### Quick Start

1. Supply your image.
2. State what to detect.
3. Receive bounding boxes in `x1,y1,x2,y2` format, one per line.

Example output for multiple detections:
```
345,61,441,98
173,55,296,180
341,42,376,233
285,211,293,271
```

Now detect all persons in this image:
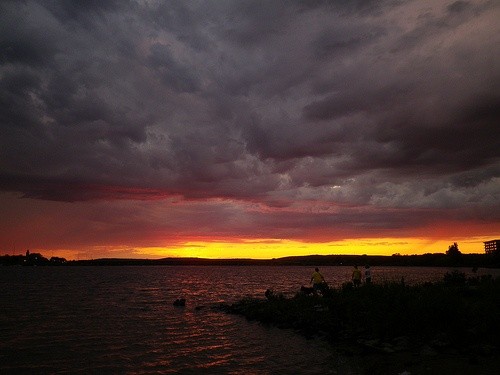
364,264,373,285
351,263,362,287
310,267,326,295
